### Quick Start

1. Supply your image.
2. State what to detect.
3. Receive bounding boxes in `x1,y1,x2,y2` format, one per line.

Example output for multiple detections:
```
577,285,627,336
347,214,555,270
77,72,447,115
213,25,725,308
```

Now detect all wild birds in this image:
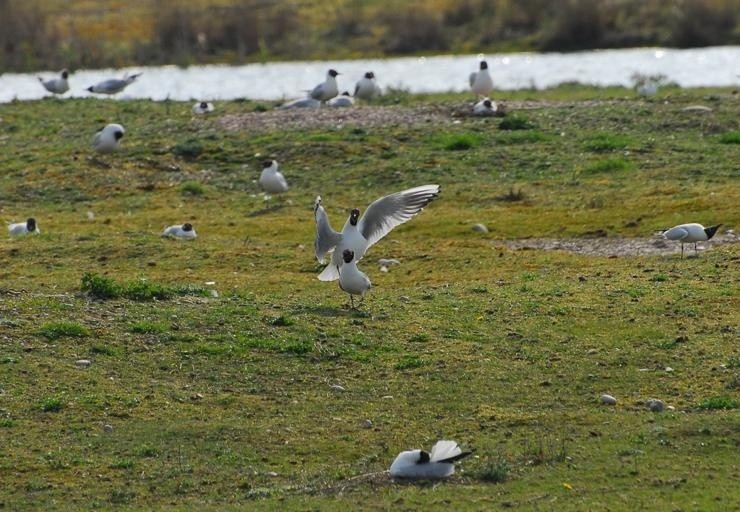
83,70,142,100
257,158,290,211
35,66,73,100
310,181,444,283
92,121,128,167
467,60,494,97
159,223,197,239
662,221,723,258
471,94,499,115
335,249,372,310
277,66,382,109
190,101,217,113
7,218,45,237
380,437,477,483
